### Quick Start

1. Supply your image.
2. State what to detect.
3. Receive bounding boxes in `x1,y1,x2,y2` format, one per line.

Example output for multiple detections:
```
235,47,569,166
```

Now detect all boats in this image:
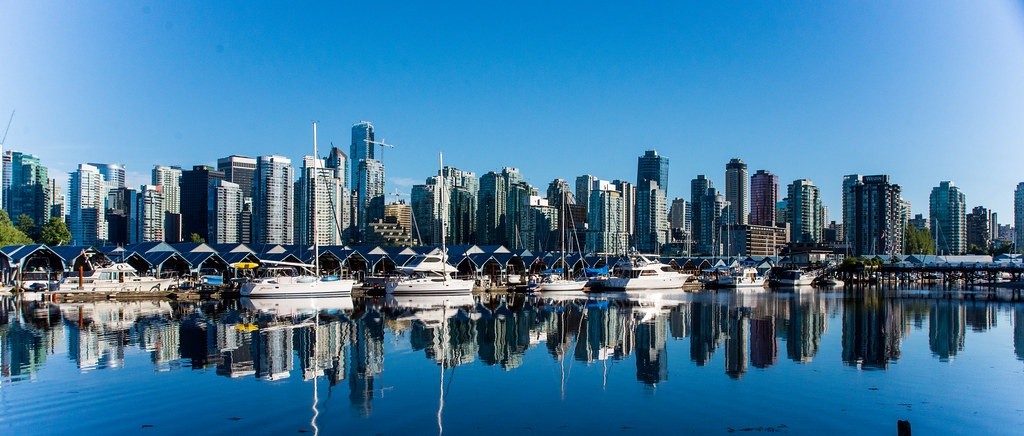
1,121,847,316
